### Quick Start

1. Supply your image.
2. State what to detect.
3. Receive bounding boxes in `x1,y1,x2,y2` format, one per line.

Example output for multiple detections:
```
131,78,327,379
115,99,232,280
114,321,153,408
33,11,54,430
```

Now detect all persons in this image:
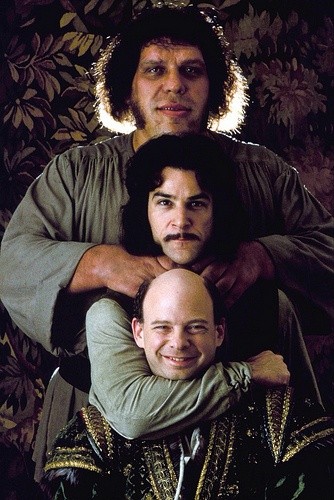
0,1,334,483
81,132,324,441
37,269,334,500
